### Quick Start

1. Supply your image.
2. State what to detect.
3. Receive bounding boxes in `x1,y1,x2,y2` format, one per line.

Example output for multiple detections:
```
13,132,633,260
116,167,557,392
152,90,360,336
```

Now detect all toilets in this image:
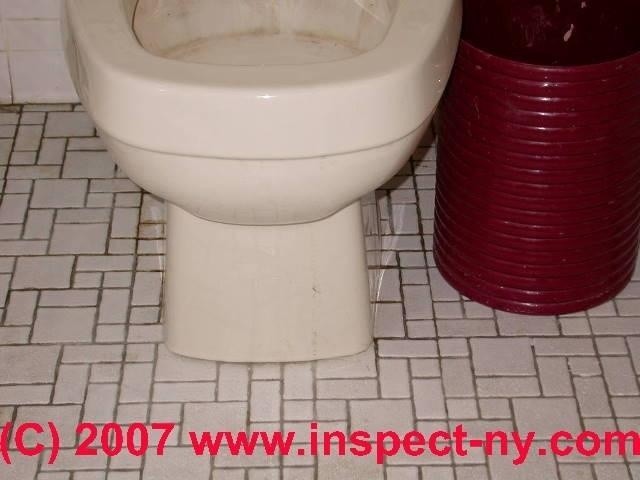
58,0,464,361
